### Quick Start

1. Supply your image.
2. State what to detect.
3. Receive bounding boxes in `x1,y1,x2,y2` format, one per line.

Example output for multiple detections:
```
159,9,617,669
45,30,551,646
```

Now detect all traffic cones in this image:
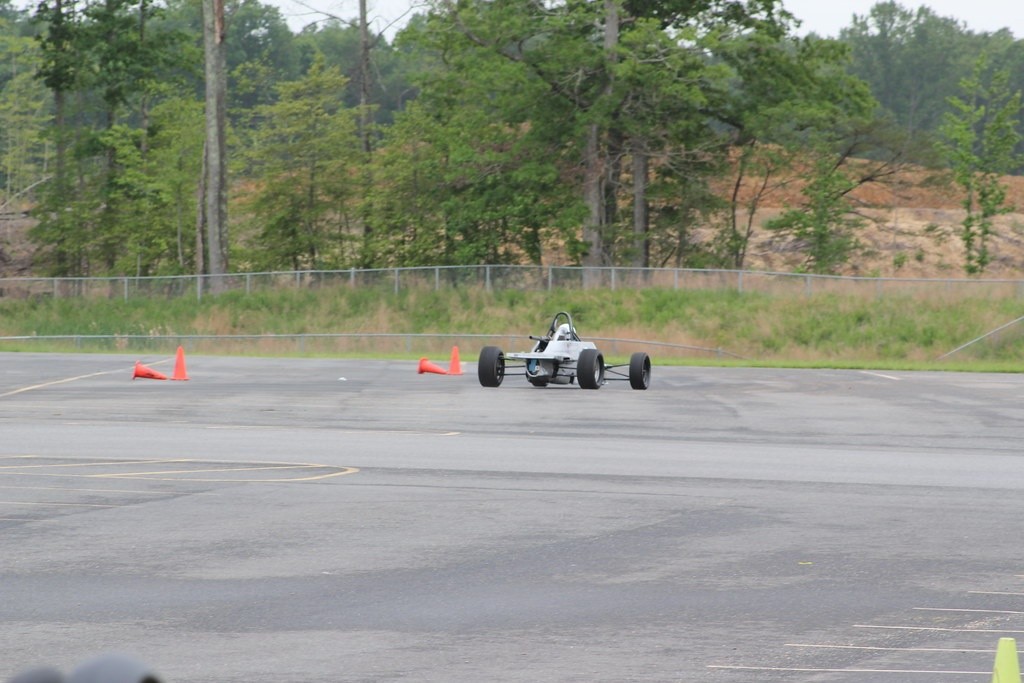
132,360,168,381
991,636,1022,683
417,357,447,375
169,346,191,381
445,345,464,375
